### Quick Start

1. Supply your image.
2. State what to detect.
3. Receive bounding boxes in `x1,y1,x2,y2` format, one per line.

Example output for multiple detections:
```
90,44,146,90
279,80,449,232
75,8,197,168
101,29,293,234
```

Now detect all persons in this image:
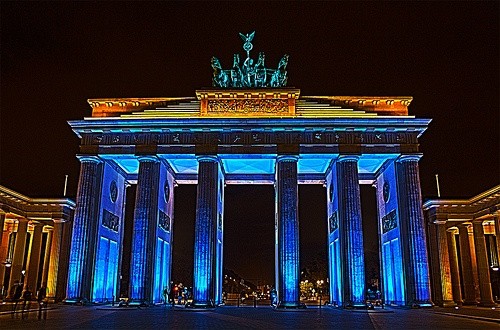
9,280,49,321
